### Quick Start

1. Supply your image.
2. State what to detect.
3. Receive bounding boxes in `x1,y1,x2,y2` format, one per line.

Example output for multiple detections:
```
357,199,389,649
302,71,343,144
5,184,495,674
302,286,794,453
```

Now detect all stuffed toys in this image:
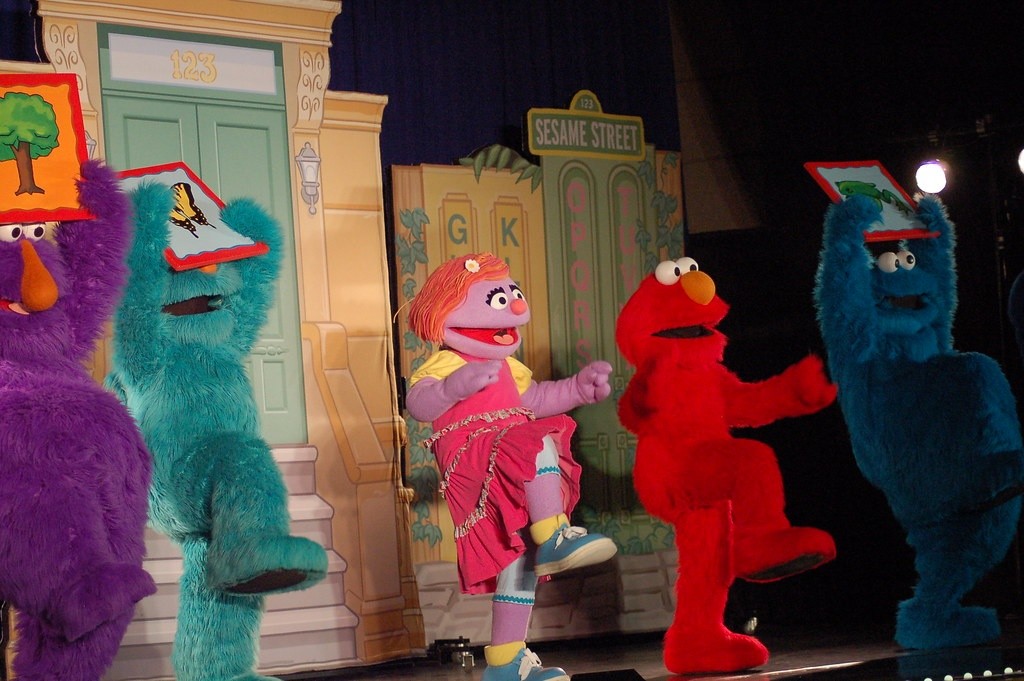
115,181,329,681
813,188,1024,650
0,162,161,681
400,248,626,680
611,255,842,670
